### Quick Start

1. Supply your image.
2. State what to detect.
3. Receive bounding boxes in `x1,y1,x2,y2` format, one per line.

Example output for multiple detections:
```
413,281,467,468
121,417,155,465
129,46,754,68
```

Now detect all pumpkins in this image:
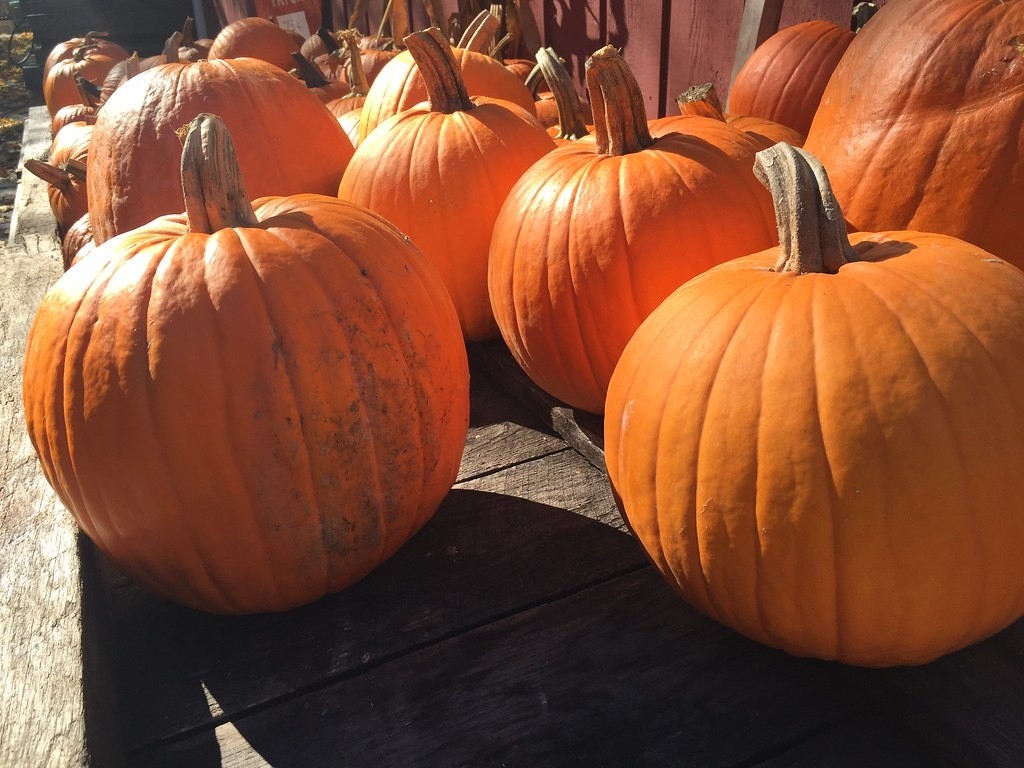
23,0,1024,669
